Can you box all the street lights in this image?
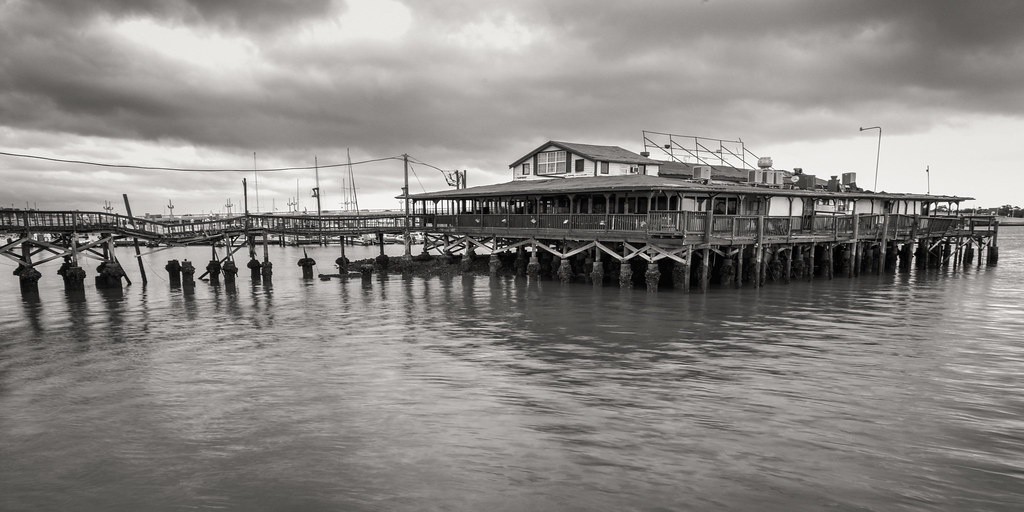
[860,126,882,231]
